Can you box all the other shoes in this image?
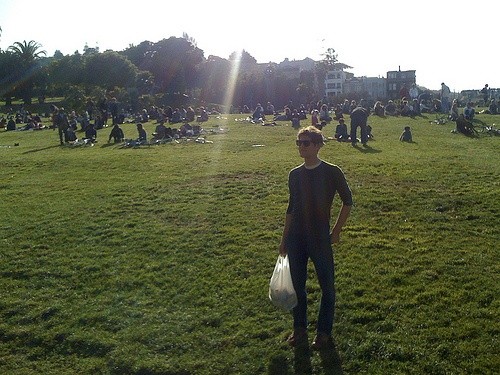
[287,332,308,345]
[312,335,329,349]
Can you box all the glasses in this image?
[296,140,312,147]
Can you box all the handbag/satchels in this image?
[269,254,298,312]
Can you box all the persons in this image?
[398,126,413,143]
[0,96,219,144]
[348,107,368,146]
[234,83,500,130]
[455,115,479,136]
[363,125,375,140]
[334,119,360,142]
[279,127,352,352]
